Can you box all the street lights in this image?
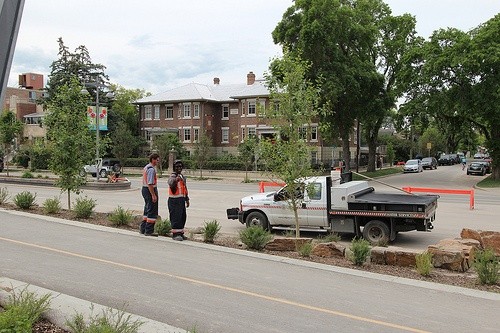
[78,75,115,180]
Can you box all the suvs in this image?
[78,157,123,178]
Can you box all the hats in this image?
[173,160,183,168]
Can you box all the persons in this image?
[167,160,190,241]
[139,154,160,237]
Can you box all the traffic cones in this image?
[259,181,266,193]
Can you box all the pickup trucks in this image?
[227,175,441,247]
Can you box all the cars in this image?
[437,151,494,174]
[403,158,423,173]
[421,156,438,170]
[467,162,487,176]
[393,160,406,166]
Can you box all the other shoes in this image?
[182,234,187,240]
[141,230,144,234]
[144,232,158,236]
[172,233,183,241]
